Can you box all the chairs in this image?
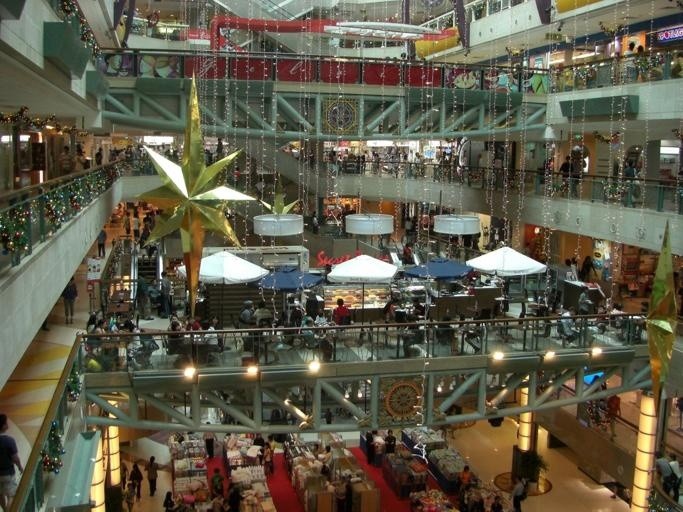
[79,280,643,373]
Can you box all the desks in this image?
[371,427,515,512]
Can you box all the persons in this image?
[62,276,78,324]
[331,475,353,512]
[365,430,396,465]
[120,454,175,511]
[623,43,648,83]
[586,64,597,89]
[623,158,638,208]
[86,271,481,371]
[325,408,333,425]
[364,151,423,175]
[211,468,244,512]
[459,464,524,511]
[605,394,621,437]
[165,149,179,165]
[2,413,23,512]
[203,422,218,459]
[58,146,142,173]
[313,442,333,465]
[405,216,418,236]
[558,156,582,198]
[312,211,319,234]
[610,480,629,504]
[654,452,682,504]
[560,254,649,345]
[123,210,159,241]
[253,433,275,474]
[216,138,223,158]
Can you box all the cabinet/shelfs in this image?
[161,424,381,512]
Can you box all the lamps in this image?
[432,215,481,238]
[344,210,394,236]
[251,210,303,237]
